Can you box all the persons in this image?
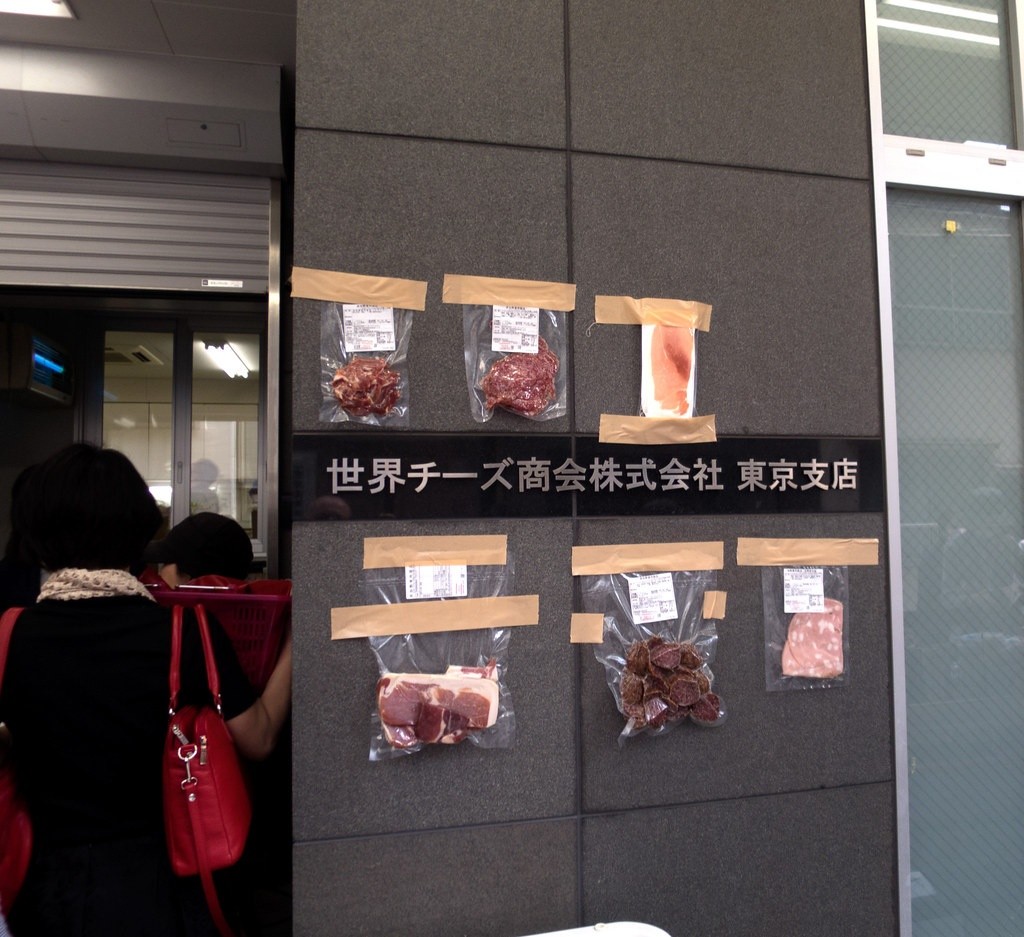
[141,512,256,608]
[0,442,294,937]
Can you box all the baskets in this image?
[149,578,292,687]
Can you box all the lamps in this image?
[204,340,250,380]
[12,323,74,408]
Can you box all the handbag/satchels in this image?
[0,605,32,919]
[164,604,251,877]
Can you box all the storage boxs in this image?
[153,579,292,688]
